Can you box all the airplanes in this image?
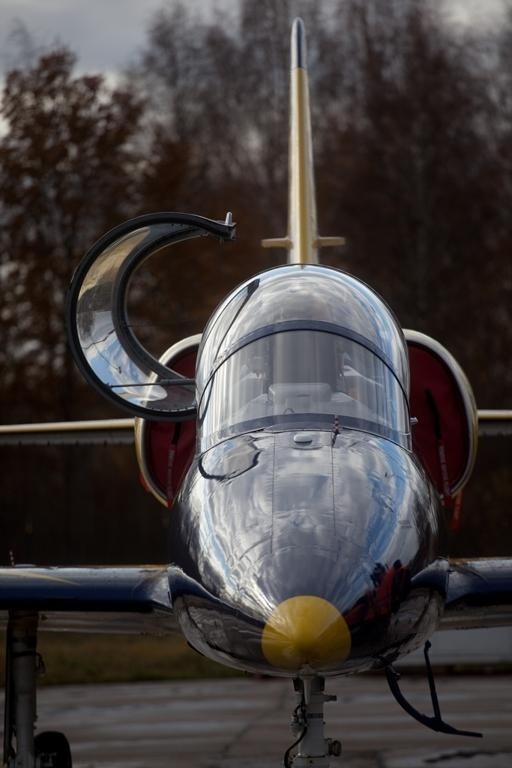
[0,16,512,767]
